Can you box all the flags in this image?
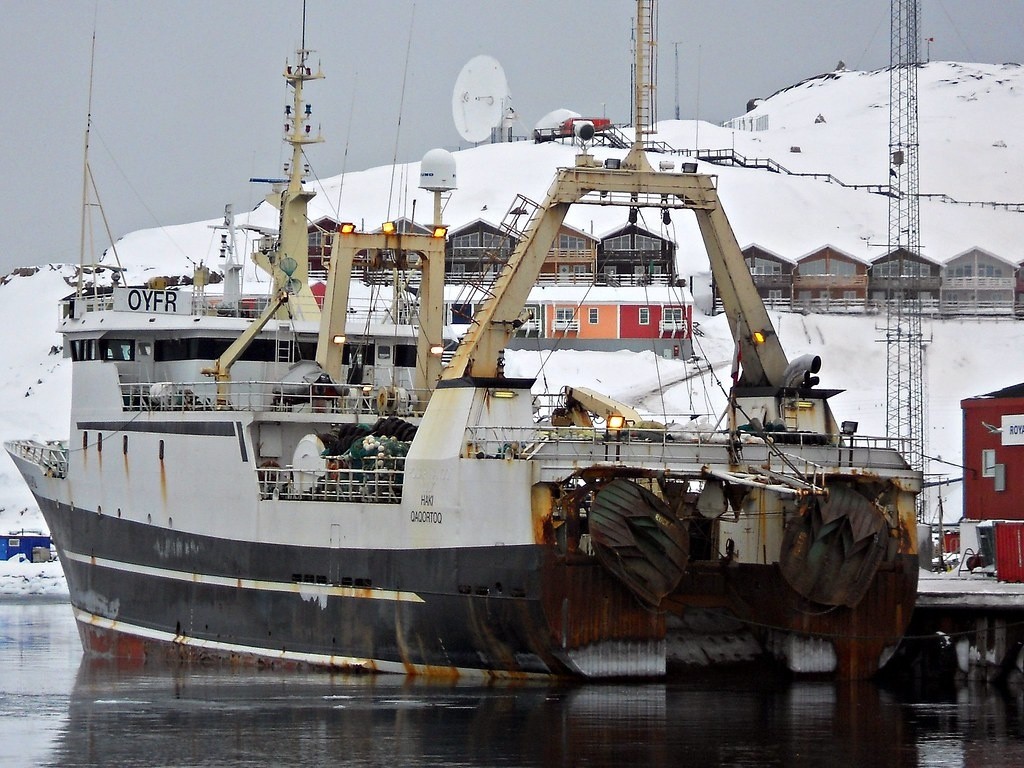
[730,315,742,386]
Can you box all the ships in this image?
[2,1,924,686]
